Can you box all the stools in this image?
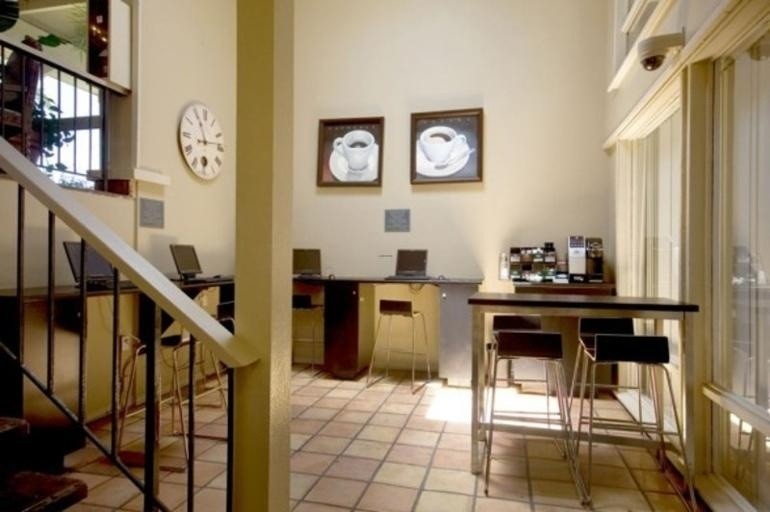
[478,326,585,506]
[569,316,666,459]
[480,316,566,460]
[573,335,699,512]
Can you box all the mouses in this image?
[329,274,335,280]
[438,275,445,282]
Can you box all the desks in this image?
[343,277,483,383]
[292,278,341,381]
[3,276,236,454]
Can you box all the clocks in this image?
[178,102,226,182]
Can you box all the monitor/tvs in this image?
[293,248,322,280]
[63,241,119,292]
[170,244,203,278]
[396,249,428,276]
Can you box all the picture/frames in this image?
[409,106,484,185]
[316,116,384,188]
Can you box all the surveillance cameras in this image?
[636,31,687,72]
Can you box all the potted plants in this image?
[30,93,76,163]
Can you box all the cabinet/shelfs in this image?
[513,278,616,394]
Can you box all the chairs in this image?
[171,301,237,409]
[0,38,42,161]
[365,300,433,393]
[293,293,325,376]
[118,327,232,473]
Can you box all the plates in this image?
[416,139,470,177]
[328,143,379,182]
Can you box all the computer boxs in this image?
[586,237,606,285]
[567,234,587,285]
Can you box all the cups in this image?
[419,126,467,168]
[333,129,375,173]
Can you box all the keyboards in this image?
[385,276,431,280]
[184,275,234,285]
[103,278,137,290]
